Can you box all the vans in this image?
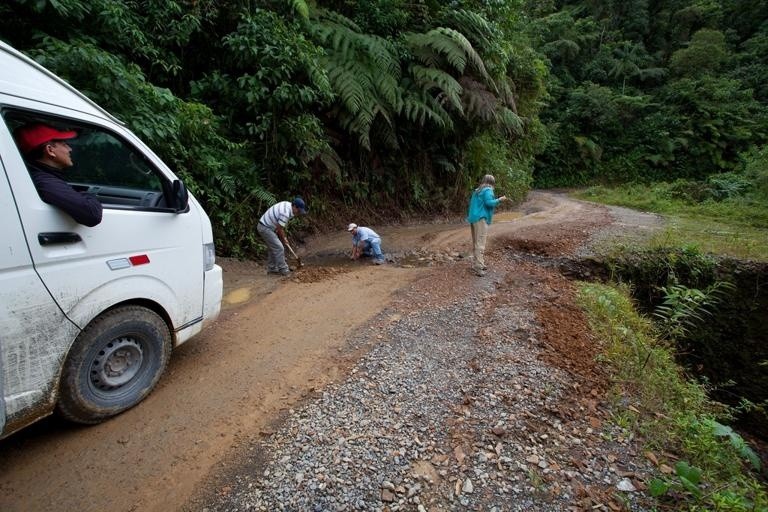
[0,40,222,441]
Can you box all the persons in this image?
[348,223,384,265]
[257,198,307,275]
[464,175,507,277]
[14,122,102,227]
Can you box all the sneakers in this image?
[376,259,384,265]
[279,268,294,275]
[267,267,280,274]
[471,265,486,277]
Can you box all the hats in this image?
[292,198,307,216]
[347,223,358,231]
[13,122,79,152]
